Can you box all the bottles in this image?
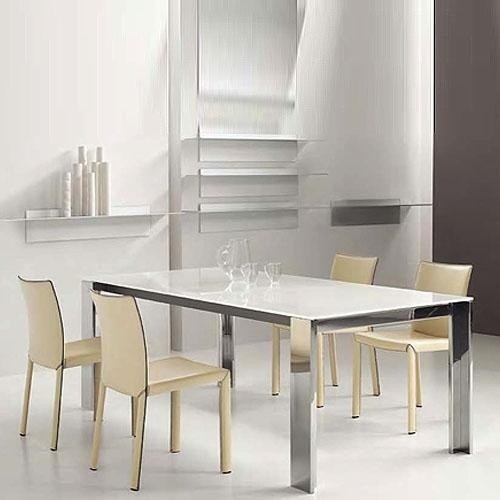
[72,145,111,216]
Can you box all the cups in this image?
[218,239,283,294]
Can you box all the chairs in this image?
[87,286,233,492]
[14,272,140,452]
[268,251,471,437]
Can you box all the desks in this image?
[80,264,481,495]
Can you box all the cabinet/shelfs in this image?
[197,133,332,215]
